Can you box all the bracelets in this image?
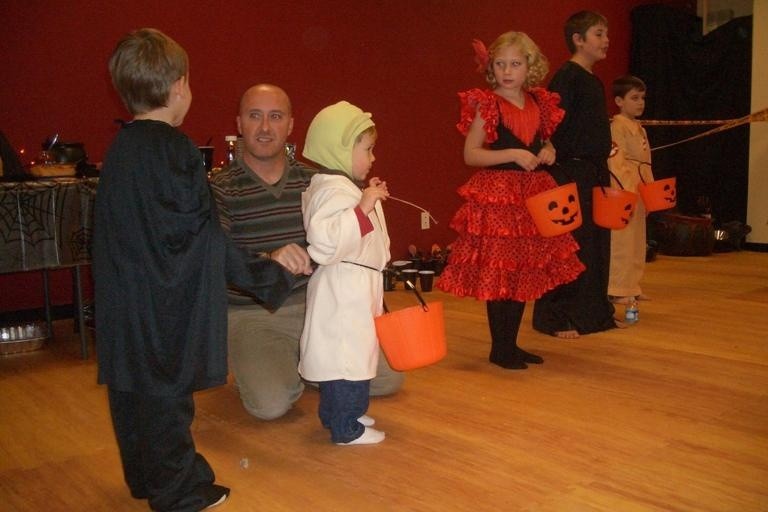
[270,250,272,259]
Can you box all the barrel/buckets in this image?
[591,167,641,234]
[519,161,584,239]
[639,158,679,214]
[375,266,450,371]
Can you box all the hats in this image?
[302,99,375,182]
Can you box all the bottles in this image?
[624,296,639,324]
[224,135,237,165]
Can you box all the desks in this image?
[0,177,98,361]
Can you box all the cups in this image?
[392,259,434,292]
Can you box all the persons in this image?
[607,74,655,305]
[435,33,588,369]
[210,84,406,420]
[531,10,628,339]
[301,101,392,446]
[91,29,296,512]
